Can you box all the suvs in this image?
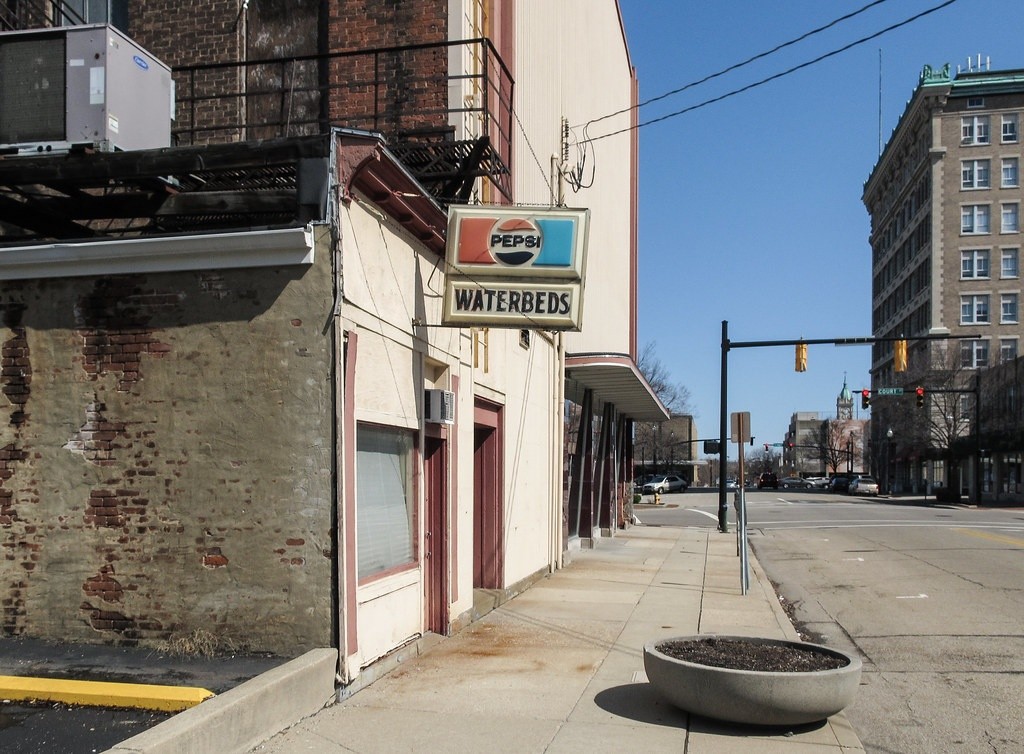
[756,473,779,490]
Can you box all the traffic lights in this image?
[915,387,924,410]
[765,444,768,451]
[789,443,792,451]
[862,389,870,410]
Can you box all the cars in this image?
[806,474,857,493]
[726,479,751,489]
[848,478,879,497]
[778,476,816,489]
[643,475,688,495]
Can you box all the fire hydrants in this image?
[654,492,661,504]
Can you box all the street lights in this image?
[886,427,894,495]
[846,439,849,473]
[670,431,674,475]
[651,424,657,477]
